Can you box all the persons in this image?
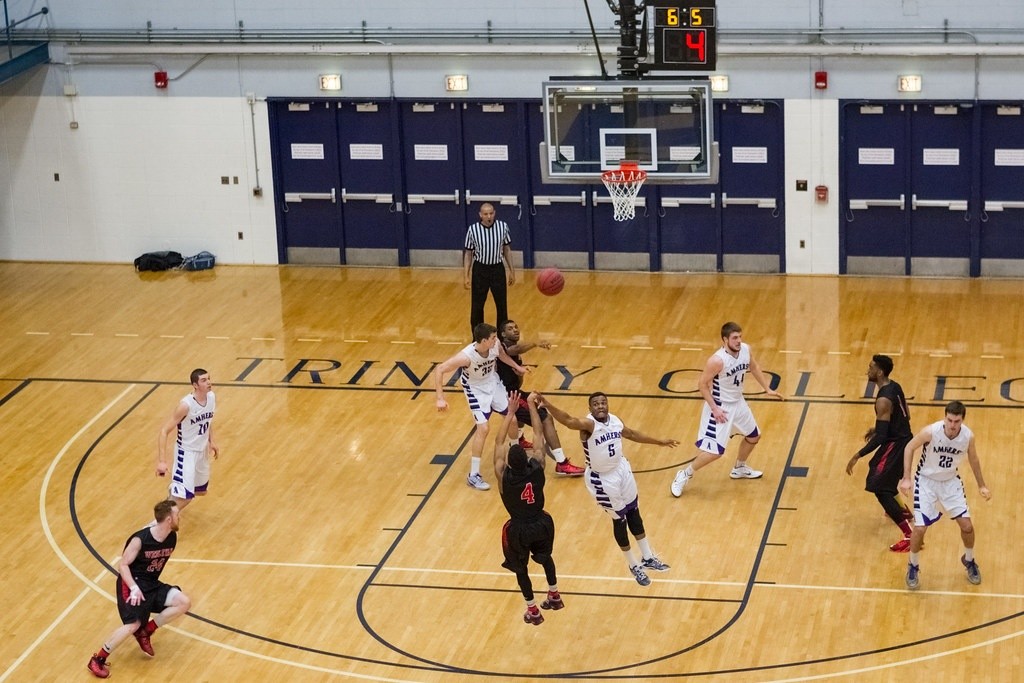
[435,323,526,490]
[146,369,218,528]
[533,389,681,586]
[493,390,566,626]
[88,500,191,679]
[845,355,925,552]
[670,321,784,497]
[495,320,585,477]
[899,401,992,589]
[463,203,516,344]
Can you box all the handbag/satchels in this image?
[182,251,216,271]
[134,251,181,271]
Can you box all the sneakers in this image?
[671,469,693,497]
[890,535,909,552]
[508,433,533,449]
[133,628,154,658]
[961,553,981,584]
[540,594,564,611]
[730,466,763,479]
[629,564,651,586]
[555,458,585,476]
[467,473,491,490]
[886,504,914,522]
[641,555,669,572]
[906,561,919,588]
[524,610,544,626]
[88,653,110,678]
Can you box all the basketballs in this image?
[537,268,565,296]
